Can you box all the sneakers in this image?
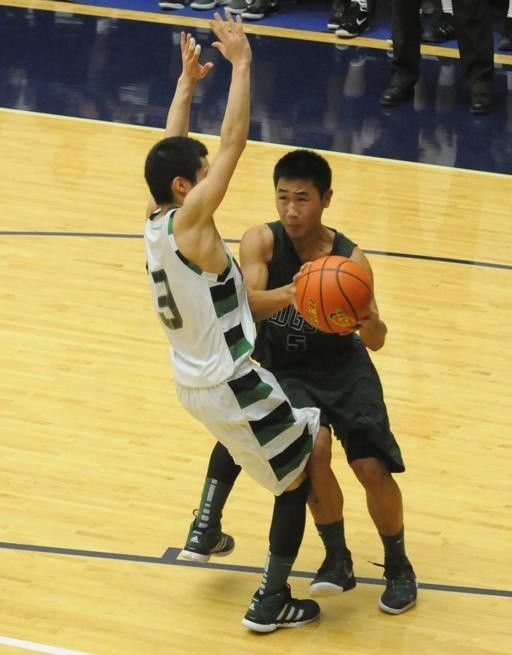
[241,584,320,632]
[378,570,417,614]
[183,520,234,560]
[421,12,457,43]
[158,0,281,19]
[327,0,371,37]
[310,549,356,593]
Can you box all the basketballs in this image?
[296,256,373,333]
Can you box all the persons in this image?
[142,10,323,636]
[237,148,422,613]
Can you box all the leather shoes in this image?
[379,85,415,105]
[468,92,496,114]
